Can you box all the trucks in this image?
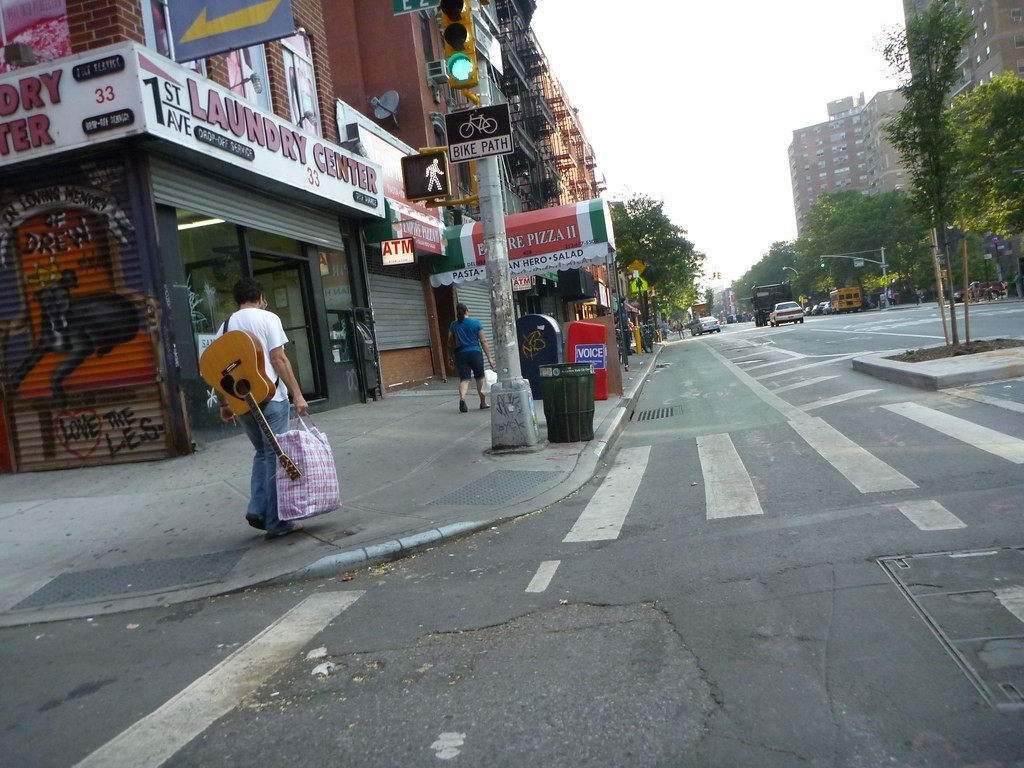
[830,287,862,314]
[692,303,711,321]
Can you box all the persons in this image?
[1012,271,1023,298]
[447,303,493,412]
[661,320,668,339]
[972,282,980,302]
[915,288,921,305]
[677,321,685,339]
[215,278,309,539]
[628,318,634,340]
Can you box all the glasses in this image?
[263,300,267,309]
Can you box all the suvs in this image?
[953,281,1006,302]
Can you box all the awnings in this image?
[427,198,629,375]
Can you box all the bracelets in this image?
[219,404,229,407]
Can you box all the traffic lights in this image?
[400,151,453,202]
[820,259,825,269]
[717,272,721,279]
[438,1,479,89]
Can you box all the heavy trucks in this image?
[749,279,794,327]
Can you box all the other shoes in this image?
[460,399,467,412]
[480,403,490,409]
[265,520,303,538]
[245,513,266,531]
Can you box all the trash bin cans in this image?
[515,313,563,400]
[538,361,596,443]
[565,320,608,400]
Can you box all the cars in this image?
[819,301,832,315]
[691,316,720,336]
[769,301,804,327]
[811,304,820,315]
[804,306,811,316]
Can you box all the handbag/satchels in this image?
[275,411,342,521]
[480,366,497,396]
[448,322,460,348]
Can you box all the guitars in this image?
[200,329,301,481]
